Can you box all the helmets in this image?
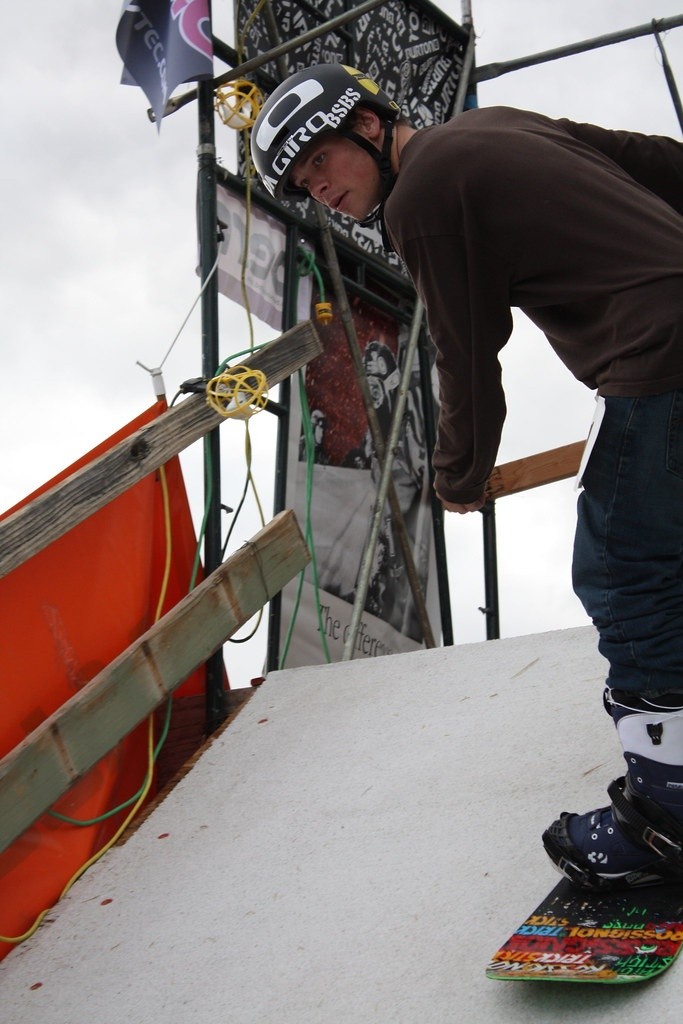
[252,63,401,203]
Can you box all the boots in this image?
[542,687,683,888]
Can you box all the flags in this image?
[116,0,213,134]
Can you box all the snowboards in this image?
[479,876,683,991]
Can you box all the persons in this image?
[251,64,683,891]
